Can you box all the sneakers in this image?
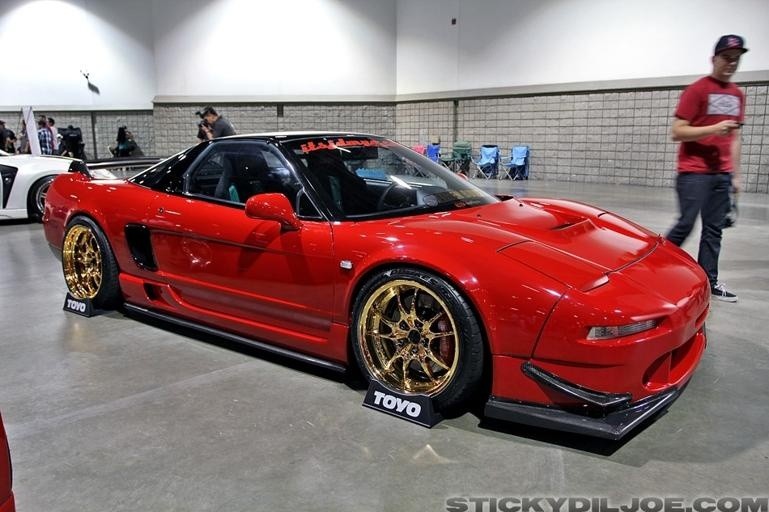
[710,284,739,303]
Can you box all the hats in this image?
[714,35,749,55]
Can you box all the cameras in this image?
[195,111,209,128]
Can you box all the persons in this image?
[662,34,750,303]
[109,126,143,158]
[199,108,237,141]
[0,115,87,162]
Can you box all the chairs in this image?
[384,140,472,179]
[224,149,284,205]
[304,146,376,220]
[499,144,531,180]
[468,144,499,179]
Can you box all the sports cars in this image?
[41,130,712,444]
[0,148,88,223]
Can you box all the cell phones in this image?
[735,121,744,126]
